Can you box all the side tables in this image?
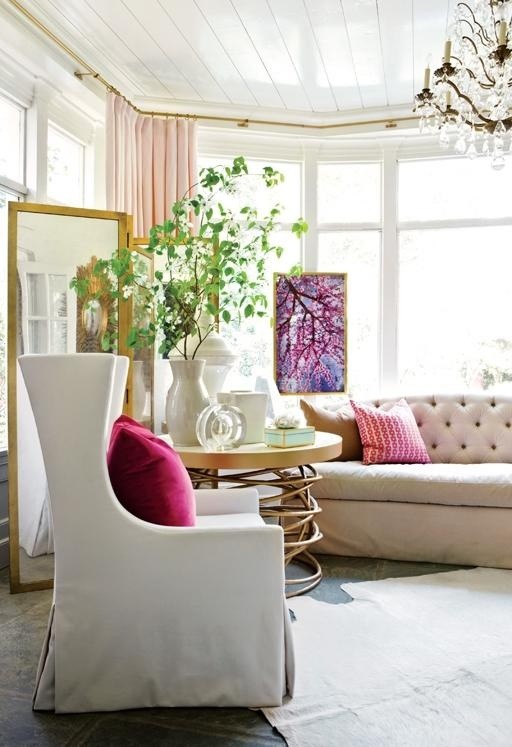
[157,429,344,599]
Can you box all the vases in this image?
[162,359,223,447]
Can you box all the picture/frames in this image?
[273,271,348,395]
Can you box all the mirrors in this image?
[7,204,133,590]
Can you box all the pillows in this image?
[350,400,431,463]
[108,415,196,528]
[298,399,362,464]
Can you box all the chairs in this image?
[18,352,293,712]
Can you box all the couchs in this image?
[288,394,511,567]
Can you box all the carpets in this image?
[248,567,512,747]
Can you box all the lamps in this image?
[410,0,512,155]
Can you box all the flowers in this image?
[68,154,309,358]
